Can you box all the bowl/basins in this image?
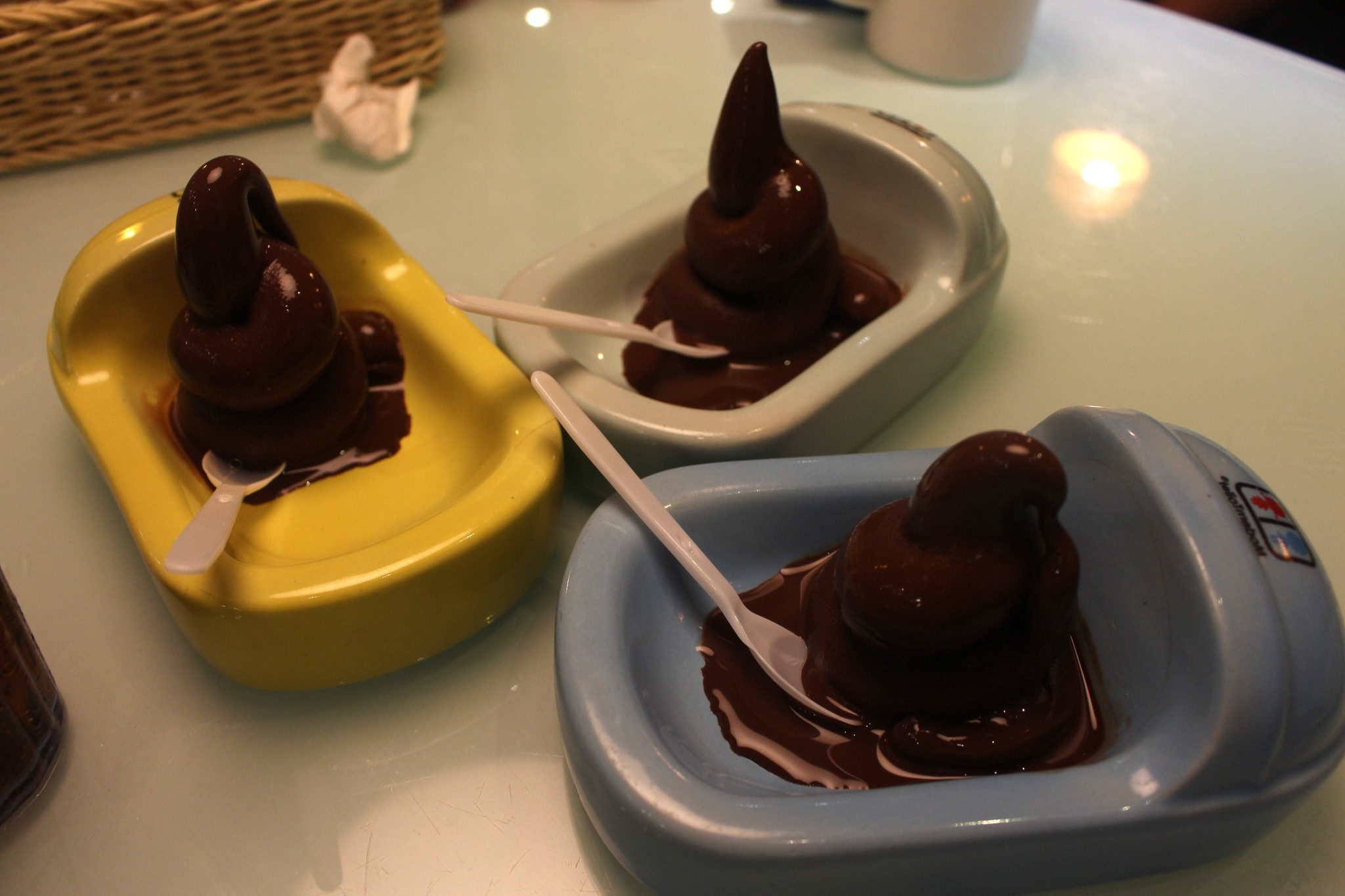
[488,102,1011,488]
[555,404,1345,896]
[41,170,563,688]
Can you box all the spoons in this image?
[532,372,860,728]
[165,452,287,578]
[447,290,727,359]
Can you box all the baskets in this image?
[0,0,450,177]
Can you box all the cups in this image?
[830,0,1037,84]
[0,572,69,829]
